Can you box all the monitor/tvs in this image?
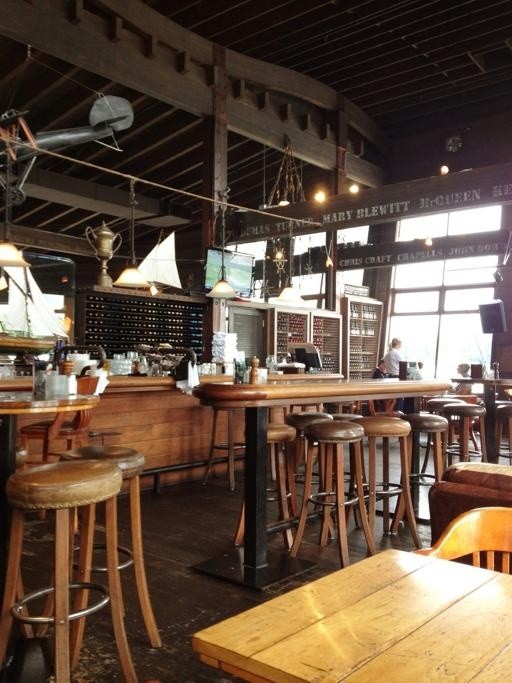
[202,246,255,298]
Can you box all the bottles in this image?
[348,301,382,381]
[277,313,307,366]
[312,314,340,374]
[84,295,204,361]
[492,361,500,380]
[36,336,95,400]
[232,354,260,385]
[13,349,34,377]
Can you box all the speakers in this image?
[479,301,507,333]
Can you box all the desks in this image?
[190,380,462,593]
[452,374,512,475]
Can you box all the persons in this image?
[373,359,391,408]
[385,337,406,411]
[449,362,472,395]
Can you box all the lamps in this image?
[205,202,239,298]
[112,178,151,289]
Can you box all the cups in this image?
[399,360,408,380]
[408,361,417,380]
[107,350,176,377]
[197,361,217,376]
[486,370,495,380]
[265,354,277,374]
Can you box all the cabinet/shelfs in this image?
[310,312,343,373]
[269,306,310,364]
[76,291,208,365]
[341,297,384,379]
